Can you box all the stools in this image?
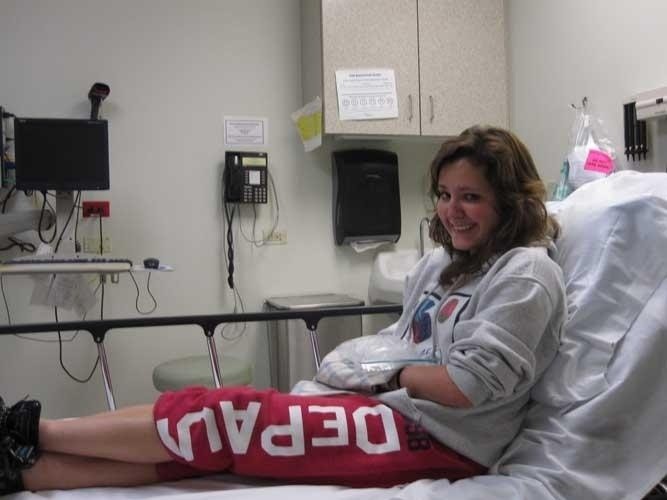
[151,354,252,394]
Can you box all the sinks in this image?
[370,251,427,304]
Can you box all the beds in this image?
[0,170,667,500]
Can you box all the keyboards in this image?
[2,257,133,267]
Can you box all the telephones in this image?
[224,150,270,206]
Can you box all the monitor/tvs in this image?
[14,116,110,191]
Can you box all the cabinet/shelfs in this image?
[300,0,512,135]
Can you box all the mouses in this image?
[143,257,160,269]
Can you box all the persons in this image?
[0,123,568,495]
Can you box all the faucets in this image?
[418,218,432,257]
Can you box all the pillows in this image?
[493,168,667,400]
